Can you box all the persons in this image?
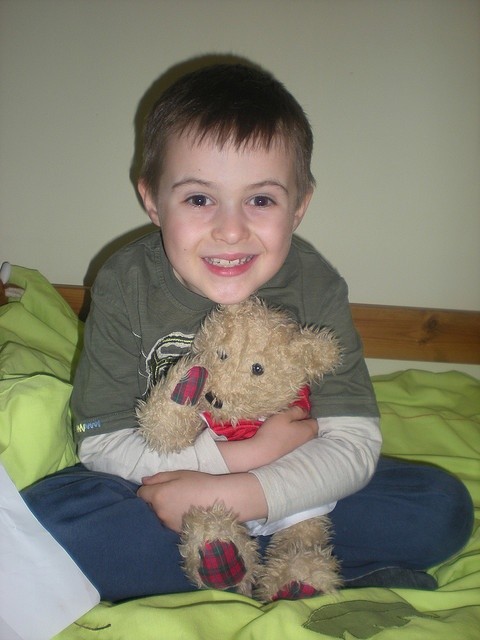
[16,50,476,609]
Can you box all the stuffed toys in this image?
[132,294,345,604]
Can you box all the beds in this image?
[0,261,480,640]
